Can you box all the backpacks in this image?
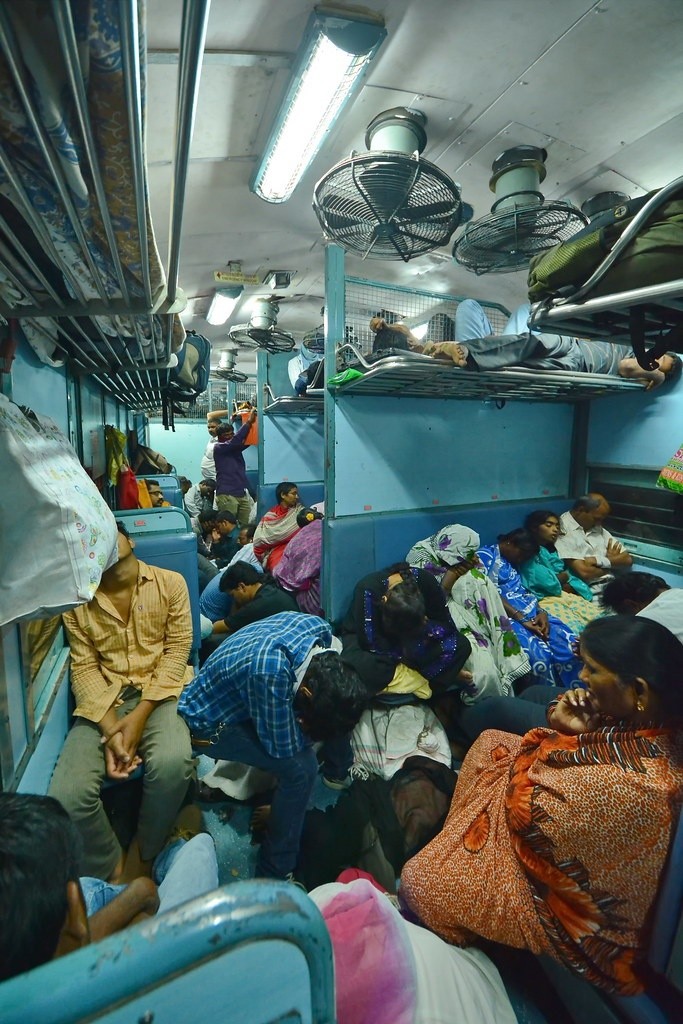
[166,330,211,401]
[296,361,324,395]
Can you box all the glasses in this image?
[224,432,234,439]
[150,491,162,496]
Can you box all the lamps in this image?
[249,3,388,205]
[206,284,244,326]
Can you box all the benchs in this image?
[245,469,324,525]
[325,494,579,632]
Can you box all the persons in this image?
[0,793,160,985]
[370,298,683,391]
[477,527,590,692]
[272,508,322,617]
[237,524,257,547]
[177,610,365,881]
[401,616,683,996]
[518,510,617,639]
[202,561,300,660]
[135,410,325,625]
[553,493,634,602]
[407,523,532,704]
[253,482,307,576]
[200,613,215,640]
[598,571,683,646]
[137,478,171,509]
[340,562,472,705]
[46,521,193,885]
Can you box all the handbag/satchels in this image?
[104,426,139,509]
[327,368,363,385]
[0,396,119,624]
[242,413,258,445]
[526,183,683,305]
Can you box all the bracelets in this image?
[512,608,548,624]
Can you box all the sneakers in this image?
[282,873,308,893]
[164,805,203,848]
[321,761,380,789]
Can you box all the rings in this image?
[574,688,580,700]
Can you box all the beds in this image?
[526,174,683,356]
[230,409,259,420]
[261,382,324,415]
[336,342,644,400]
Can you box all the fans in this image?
[311,106,462,263]
[209,349,248,384]
[452,144,592,276]
[300,306,363,356]
[226,298,295,355]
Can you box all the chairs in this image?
[134,474,183,508]
[112,506,201,779]
[0,879,337,1024]
